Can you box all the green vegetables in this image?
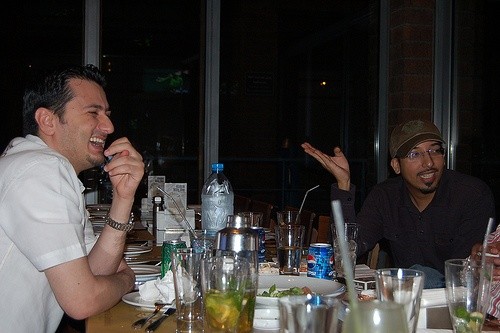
[455,307,482,333]
[225,275,305,328]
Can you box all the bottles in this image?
[201,163,234,230]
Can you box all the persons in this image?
[302,119,496,289]
[469,241,500,283]
[0,63,145,333]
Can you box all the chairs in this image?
[233,194,328,242]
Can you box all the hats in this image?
[388,119,443,159]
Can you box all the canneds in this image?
[306,242,335,282]
[161,238,188,278]
[248,226,265,262]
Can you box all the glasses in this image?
[394,147,444,161]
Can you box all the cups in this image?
[171,210,359,333]
[278,258,494,333]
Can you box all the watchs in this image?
[105,209,136,233]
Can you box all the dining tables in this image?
[86,237,345,333]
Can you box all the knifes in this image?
[145,300,176,333]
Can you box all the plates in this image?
[123,246,153,254]
[256,275,345,305]
[91,220,107,227]
[122,292,197,308]
[129,265,162,278]
